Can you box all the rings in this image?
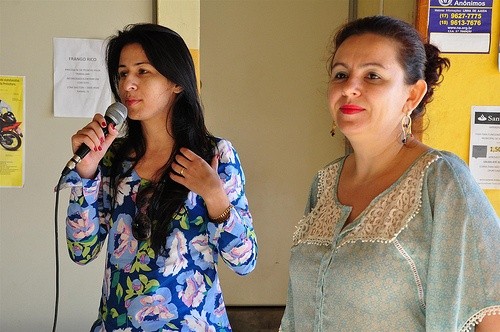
[180,167,186,177]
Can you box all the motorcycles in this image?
[0,111,22,151]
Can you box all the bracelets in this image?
[208,205,234,223]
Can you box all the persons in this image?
[57,22,258,331]
[275,16,500,332]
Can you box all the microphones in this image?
[61,101,128,175]
